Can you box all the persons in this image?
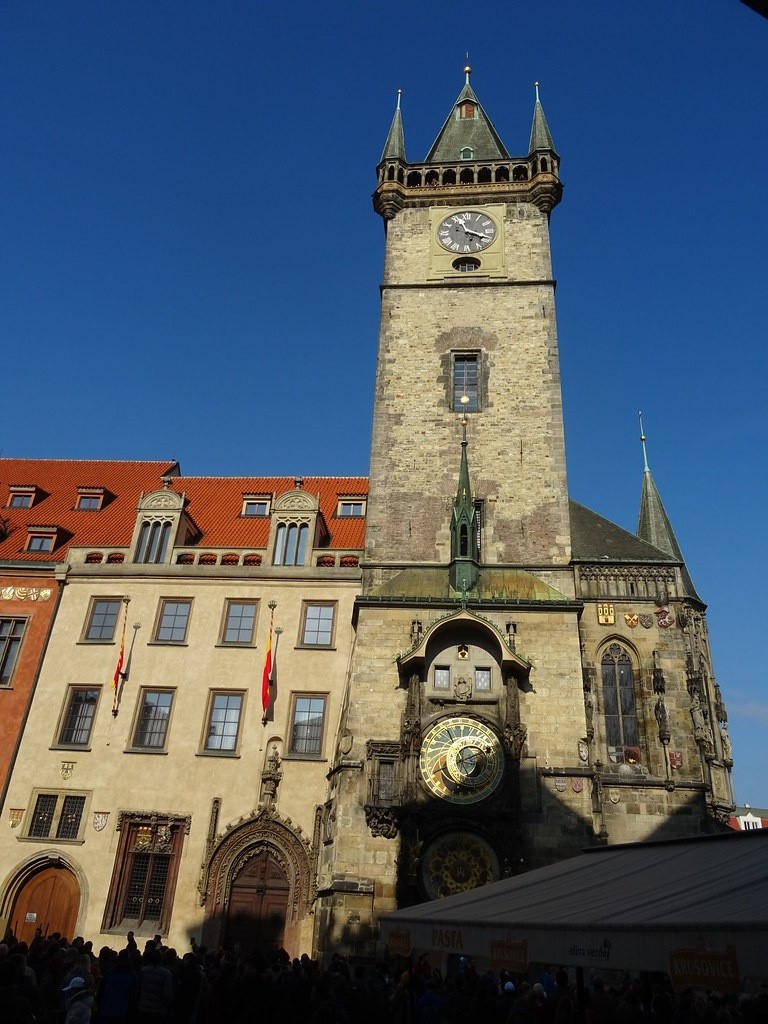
[0,925,768,1024]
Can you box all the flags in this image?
[262,626,272,717]
[113,605,128,697]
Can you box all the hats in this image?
[533,983,544,993]
[504,981,515,992]
[61,976,85,991]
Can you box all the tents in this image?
[376,826,767,986]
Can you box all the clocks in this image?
[434,207,500,254]
